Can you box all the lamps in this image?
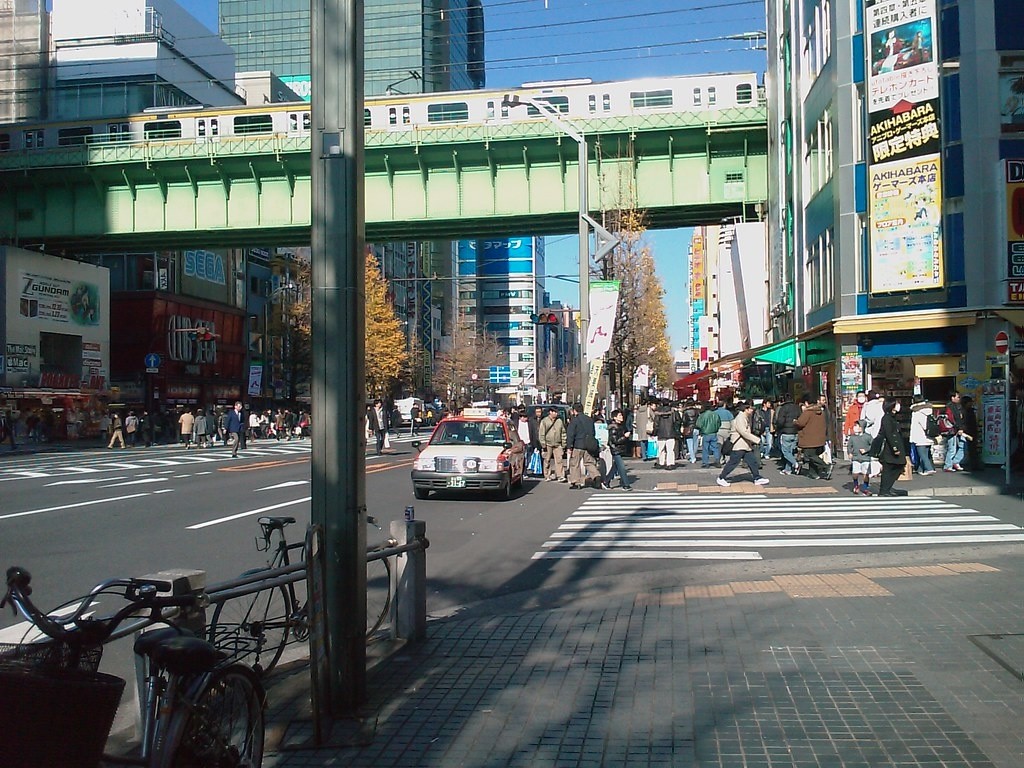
[22,243,46,252]
[46,248,67,257]
[67,255,84,263]
[719,215,743,246]
[95,258,103,268]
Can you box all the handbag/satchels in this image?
[212,424,217,432]
[531,448,543,474]
[586,435,599,455]
[910,443,919,464]
[721,438,732,455]
[648,436,659,458]
[384,432,391,449]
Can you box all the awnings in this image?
[710,337,796,373]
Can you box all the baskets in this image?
[0,640,125,768]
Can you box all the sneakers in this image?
[754,477,770,486]
[716,477,731,486]
[862,490,873,496]
[853,484,859,494]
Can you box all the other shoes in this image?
[807,475,821,479]
[826,464,834,479]
[524,473,633,492]
[878,490,898,496]
[377,451,382,455]
[780,469,792,476]
[795,463,802,475]
[641,454,771,470]
[915,463,963,475]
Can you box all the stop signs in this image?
[995,331,1009,354]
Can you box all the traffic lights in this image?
[530,313,558,325]
[188,331,215,341]
[602,363,609,375]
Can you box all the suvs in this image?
[525,404,571,427]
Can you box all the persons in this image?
[909,394,936,475]
[844,391,867,474]
[847,420,874,496]
[249,407,311,443]
[448,404,633,491]
[0,409,20,451]
[860,390,885,439]
[366,399,423,456]
[943,389,968,472]
[876,396,906,497]
[1001,382,1024,471]
[911,31,925,63]
[882,26,903,58]
[960,396,984,470]
[632,396,834,487]
[26,409,226,450]
[223,401,245,457]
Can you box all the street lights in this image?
[504,95,621,408]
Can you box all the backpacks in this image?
[919,410,940,437]
[939,403,955,437]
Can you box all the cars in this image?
[410,415,526,498]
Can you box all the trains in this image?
[0,70,766,169]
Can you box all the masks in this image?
[858,397,866,403]
[894,404,900,412]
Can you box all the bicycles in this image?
[209,515,392,677]
[0,566,267,768]
